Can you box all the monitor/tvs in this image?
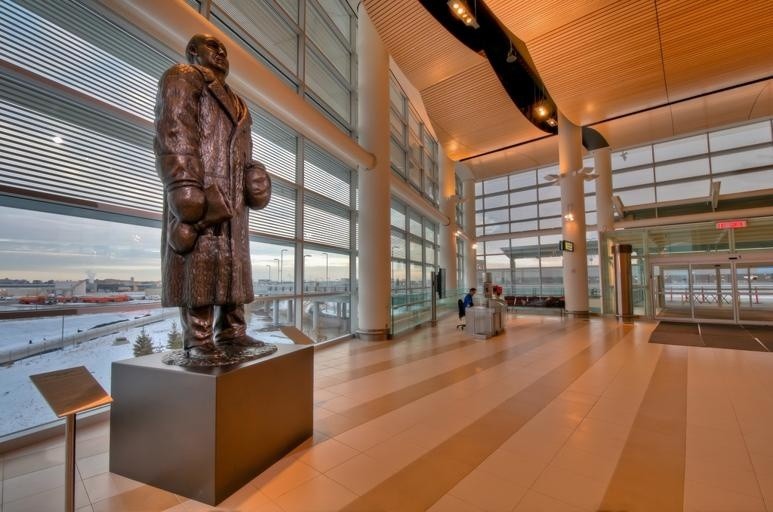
[437,268,448,299]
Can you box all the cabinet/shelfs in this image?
[467,302,506,340]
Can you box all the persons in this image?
[154,33,270,361]
[464,287,477,309]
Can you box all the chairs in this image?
[457,299,466,331]
[504,296,565,318]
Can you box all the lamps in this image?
[542,167,600,185]
[447,0,477,26]
[532,86,549,120]
